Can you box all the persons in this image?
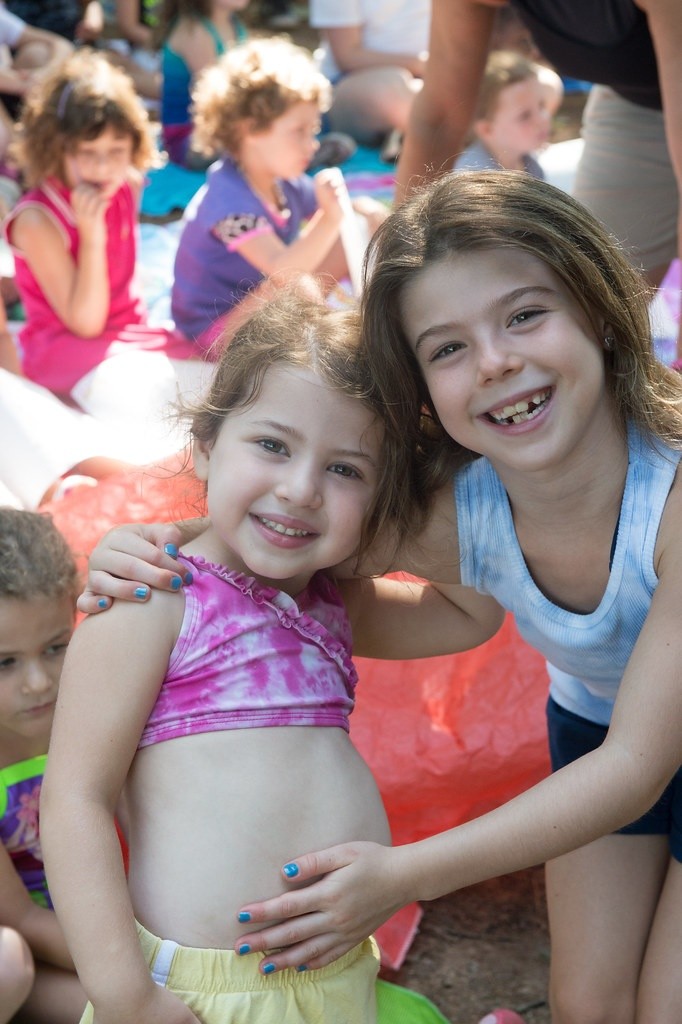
[0,2,680,390]
[0,509,72,1024]
[76,170,682,1024]
[35,304,501,1024]
